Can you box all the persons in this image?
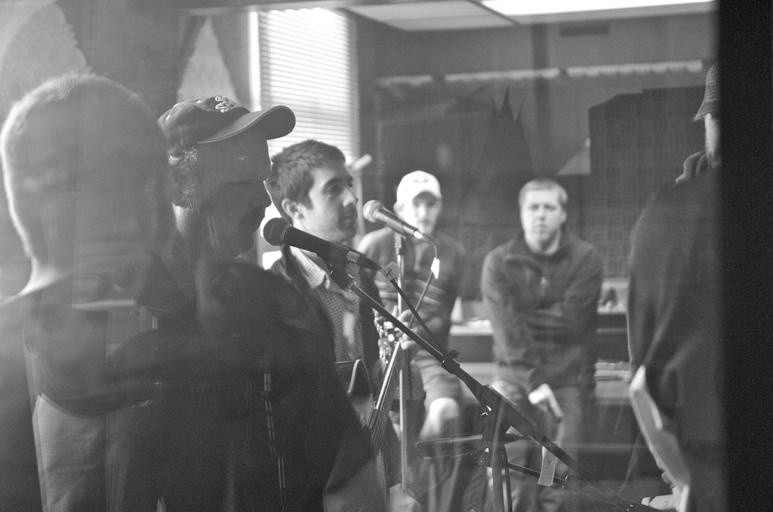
[258,137,403,512]
[155,95,297,261]
[344,170,465,511]
[0,72,392,511]
[624,60,724,511]
[477,177,602,511]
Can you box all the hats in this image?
[157,95,296,145]
[689,61,724,125]
[397,171,441,205]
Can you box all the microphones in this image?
[364,199,430,244]
[261,218,381,276]
